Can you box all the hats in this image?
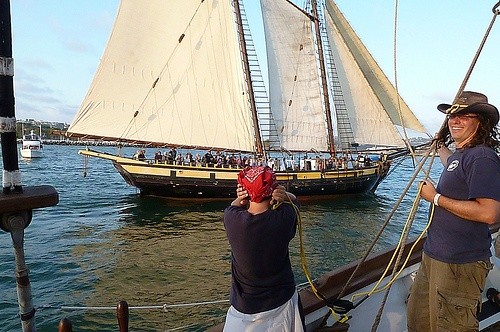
[437,91,500,127]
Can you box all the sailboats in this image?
[64,0,428,198]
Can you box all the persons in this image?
[221,165,307,332]
[407,90,500,332]
[133,148,372,174]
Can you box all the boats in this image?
[19,131,44,158]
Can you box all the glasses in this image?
[446,115,478,120]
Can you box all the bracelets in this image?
[434,194,442,206]
